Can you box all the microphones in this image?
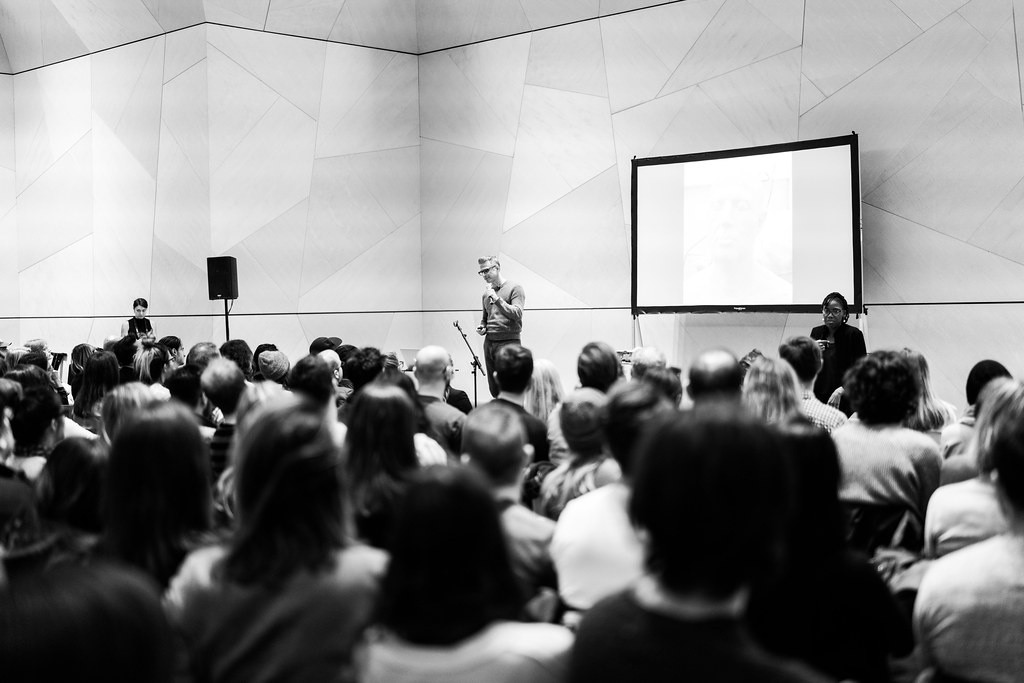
[487,286,494,305]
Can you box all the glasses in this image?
[822,308,844,316]
[340,360,345,367]
[478,265,495,275]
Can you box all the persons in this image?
[121,298,155,341]
[0,338,1023,682]
[809,292,866,420]
[476,255,524,398]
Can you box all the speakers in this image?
[206,256,239,300]
